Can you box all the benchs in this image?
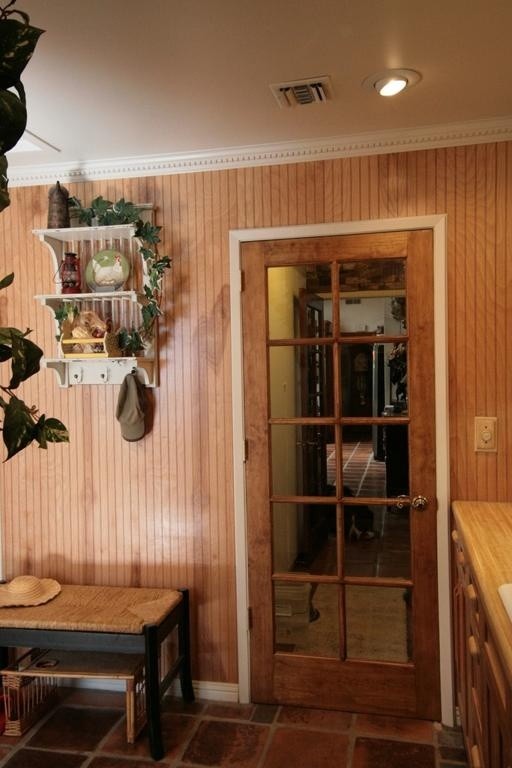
[0,647,149,743]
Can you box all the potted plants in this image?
[76,200,165,353]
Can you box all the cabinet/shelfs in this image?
[383,404,412,510]
[447,501,512,762]
[29,200,159,386]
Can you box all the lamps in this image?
[361,69,423,97]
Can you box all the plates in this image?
[86,250,129,292]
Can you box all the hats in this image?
[118,374,148,442]
[2,577,62,607]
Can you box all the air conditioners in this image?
[270,75,337,109]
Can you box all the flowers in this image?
[387,344,407,360]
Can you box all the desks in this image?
[0,582,198,759]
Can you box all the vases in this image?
[392,397,412,409]
